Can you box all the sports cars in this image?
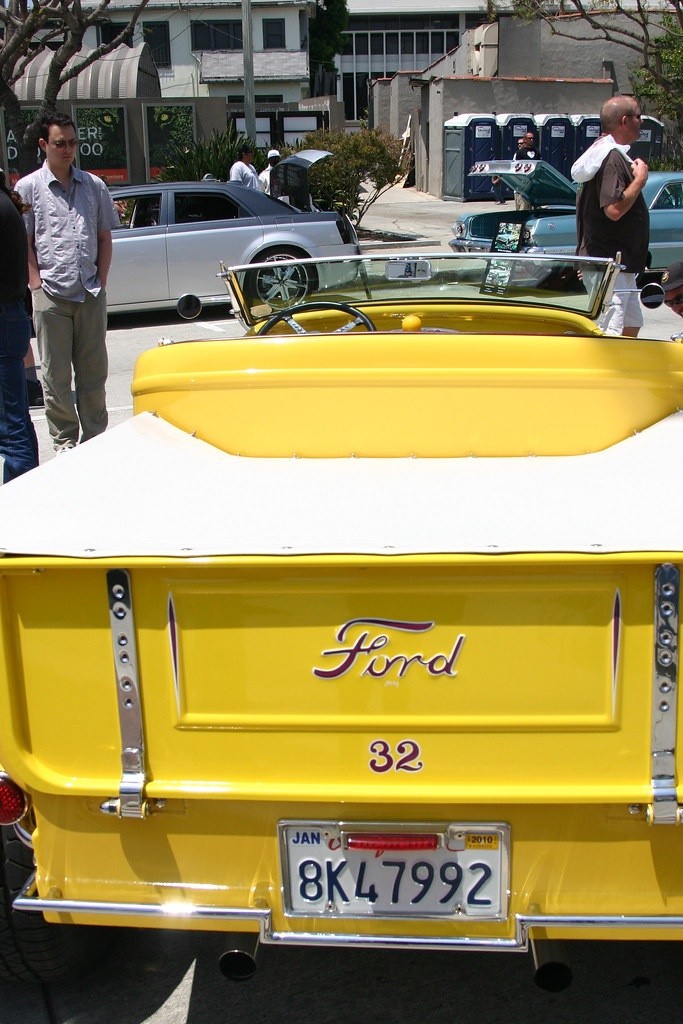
[0,250,683,992]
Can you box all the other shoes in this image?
[27,378,42,402]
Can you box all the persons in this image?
[258,149,290,205]
[511,131,541,210]
[0,167,44,483]
[229,145,260,191]
[491,175,507,205]
[570,96,650,338]
[12,112,120,457]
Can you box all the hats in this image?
[268,149,281,159]
[661,262,683,291]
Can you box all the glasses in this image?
[627,114,642,119]
[517,143,523,145]
[526,137,535,140]
[664,294,683,307]
[48,138,79,148]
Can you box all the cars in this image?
[22,148,364,336]
[448,157,683,291]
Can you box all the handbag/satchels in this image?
[570,134,635,183]
